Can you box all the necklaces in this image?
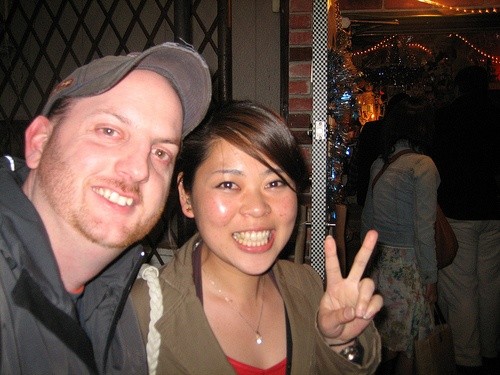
[203,269,269,345]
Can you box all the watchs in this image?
[325,341,362,363]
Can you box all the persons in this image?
[435,66,500,375]
[129,100,383,375]
[361,115,458,375]
[0,44,212,375]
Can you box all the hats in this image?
[42,42,213,140]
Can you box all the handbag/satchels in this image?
[433,203,458,269]
